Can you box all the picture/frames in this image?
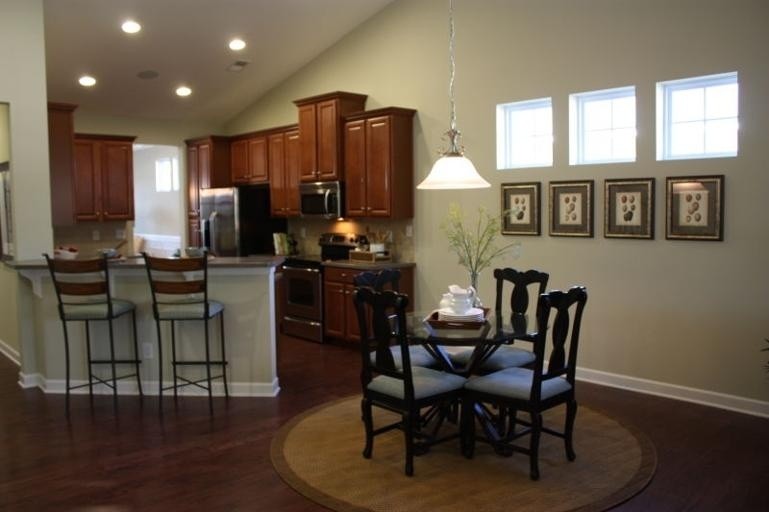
[664,174,724,242]
[500,183,540,237]
[604,178,654,241]
[548,179,595,238]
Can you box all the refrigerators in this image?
[199,184,273,259]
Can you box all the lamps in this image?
[415,0,493,190]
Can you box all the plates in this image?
[436,307,485,325]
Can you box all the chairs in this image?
[352,286,468,478]
[463,285,588,480]
[351,267,459,434]
[141,251,227,416]
[43,253,143,412]
[492,267,549,437]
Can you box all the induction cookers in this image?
[285,232,366,262]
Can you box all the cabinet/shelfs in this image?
[292,90,369,182]
[183,134,232,248]
[321,259,415,352]
[73,135,140,222]
[47,101,80,227]
[341,106,418,223]
[229,129,269,184]
[268,121,299,218]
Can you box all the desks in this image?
[383,307,531,458]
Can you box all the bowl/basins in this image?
[52,249,80,262]
[184,245,208,257]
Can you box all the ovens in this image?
[275,258,326,344]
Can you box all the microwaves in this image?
[298,180,347,220]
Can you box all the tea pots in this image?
[448,283,478,313]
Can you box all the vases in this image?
[469,271,481,294]
